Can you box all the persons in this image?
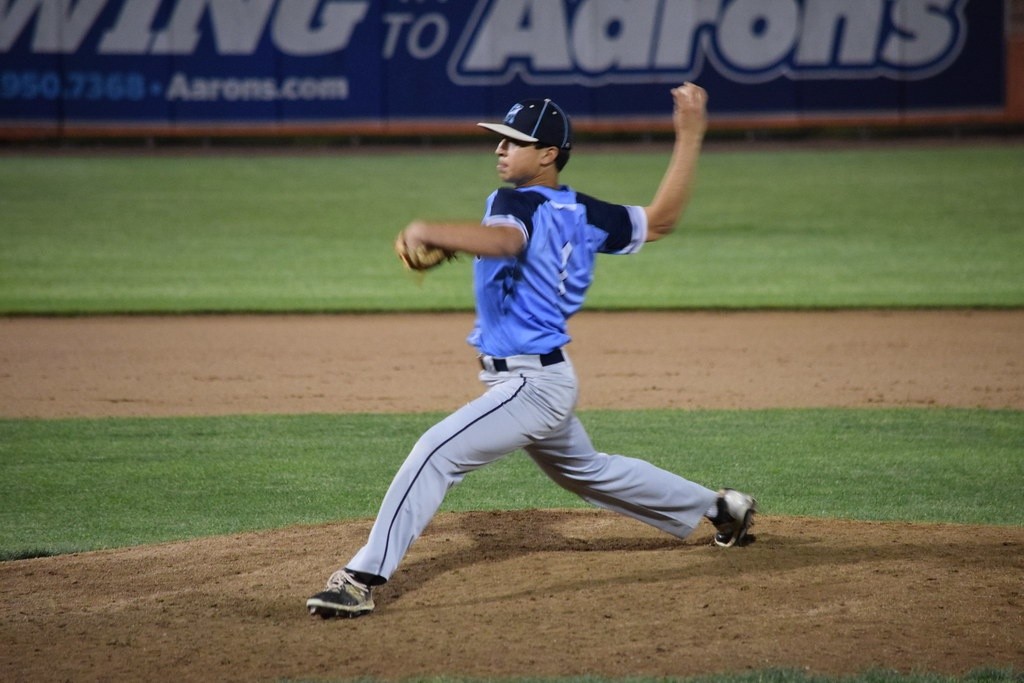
[305,81,758,620]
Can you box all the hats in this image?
[476,98,571,149]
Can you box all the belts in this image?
[477,349,564,373]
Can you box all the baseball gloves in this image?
[394,227,459,272]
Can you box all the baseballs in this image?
[676,85,702,103]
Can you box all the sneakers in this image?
[305,570,375,619]
[705,487,757,547]
